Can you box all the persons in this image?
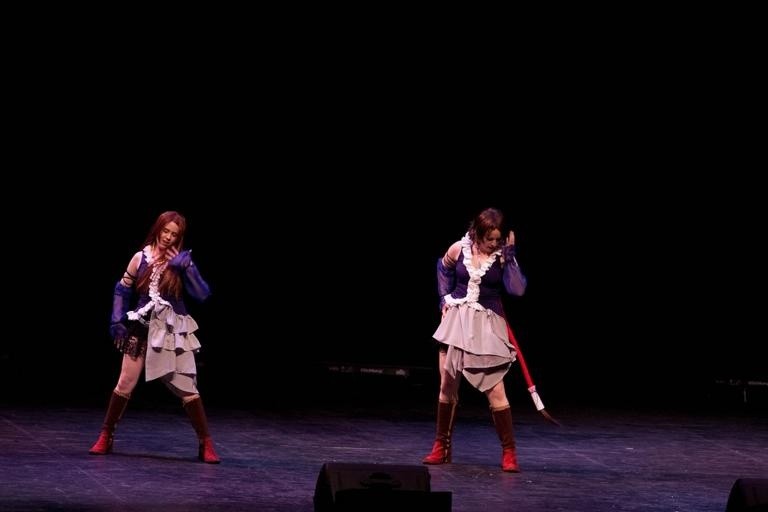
[88,209,222,465]
[420,209,530,473]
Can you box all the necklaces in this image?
[152,242,168,264]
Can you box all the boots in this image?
[420,397,459,466]
[489,403,521,474]
[88,389,132,456]
[182,394,219,464]
